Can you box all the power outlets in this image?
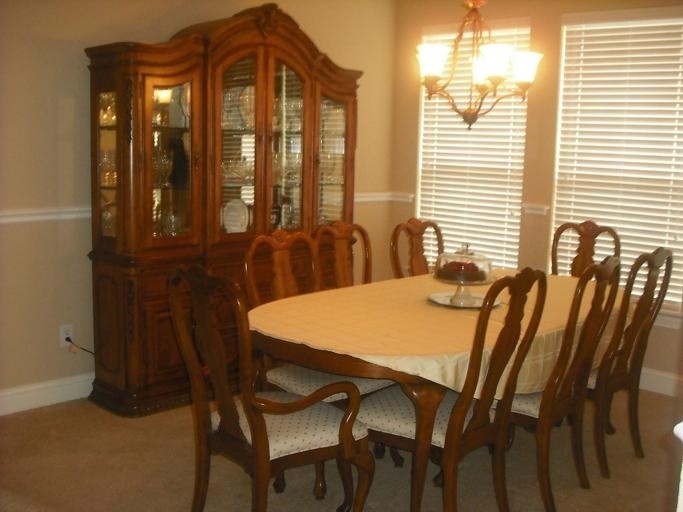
[57,324,75,348]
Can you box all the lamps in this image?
[415,0,545,129]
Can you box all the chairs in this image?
[350,267,547,510]
[244,232,404,498]
[571,246,674,477]
[170,265,374,511]
[552,219,622,274]
[314,219,374,291]
[480,255,627,511]
[390,219,446,278]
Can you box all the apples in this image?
[442,260,479,273]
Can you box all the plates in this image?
[428,293,501,310]
[221,197,249,233]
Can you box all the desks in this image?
[245,271,640,511]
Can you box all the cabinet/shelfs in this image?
[84,2,364,415]
